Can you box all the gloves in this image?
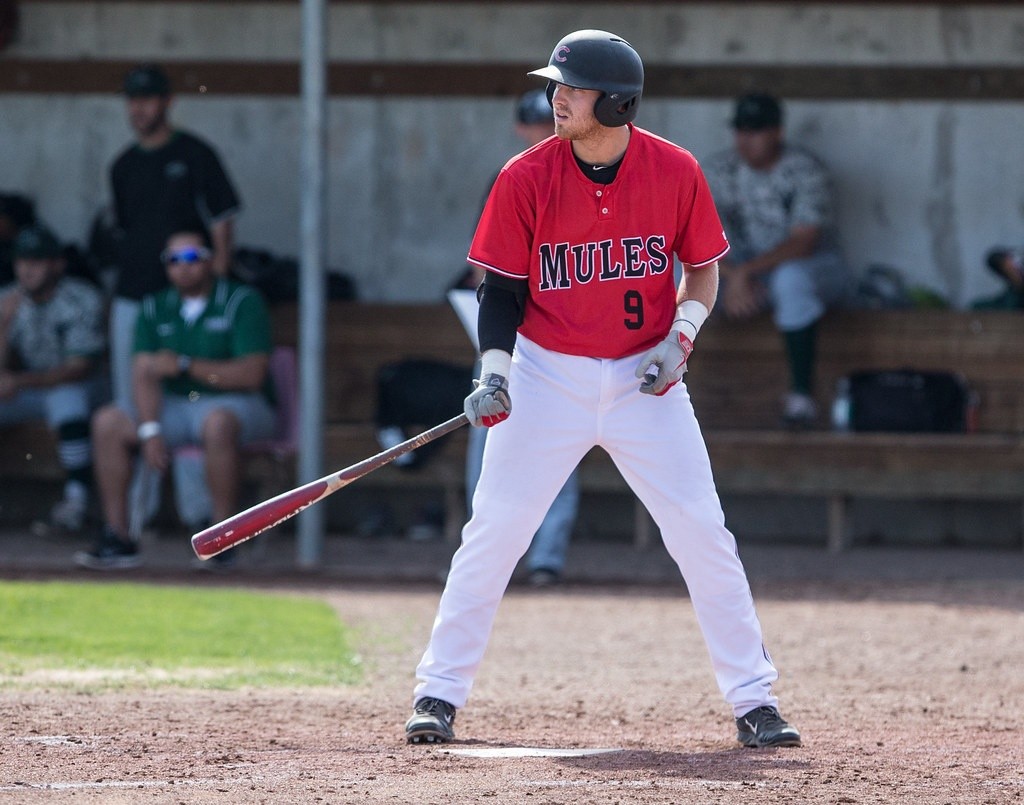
[635,330,694,396]
[464,372,512,427]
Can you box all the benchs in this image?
[270,301,1023,556]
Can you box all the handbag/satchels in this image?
[833,369,968,432]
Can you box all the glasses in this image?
[166,250,206,264]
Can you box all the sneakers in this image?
[736,706,802,747]
[406,697,456,742]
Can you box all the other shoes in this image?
[783,394,818,421]
[531,569,556,586]
[76,534,146,570]
[192,548,236,572]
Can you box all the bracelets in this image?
[137,424,161,440]
[178,354,192,379]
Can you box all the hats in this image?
[15,227,60,261]
[118,67,166,97]
[732,94,779,129]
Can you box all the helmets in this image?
[527,30,644,127]
[517,88,554,125]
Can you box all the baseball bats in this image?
[191,388,510,563]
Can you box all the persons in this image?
[407,30,800,747]
[466,91,578,586]
[0,65,283,572]
[700,93,844,423]
